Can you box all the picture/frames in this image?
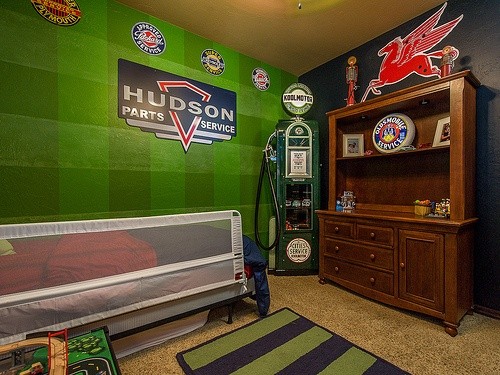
[343,133,364,158]
[430,116,450,147]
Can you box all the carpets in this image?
[175,307,411,375]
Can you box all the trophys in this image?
[439,45,453,78]
[344,55,360,107]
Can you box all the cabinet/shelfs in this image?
[399,220,475,336]
[325,69,481,220]
[315,210,399,307]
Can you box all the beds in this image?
[1,210,271,359]
